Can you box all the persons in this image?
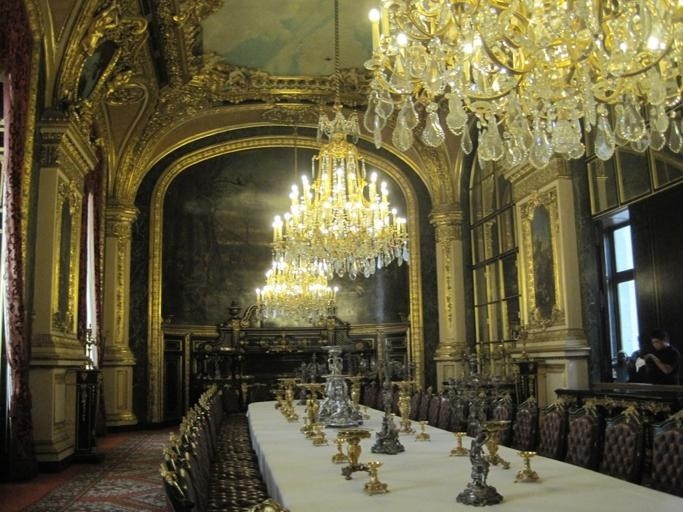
[610,352,631,383]
[628,333,653,381]
[640,328,681,386]
